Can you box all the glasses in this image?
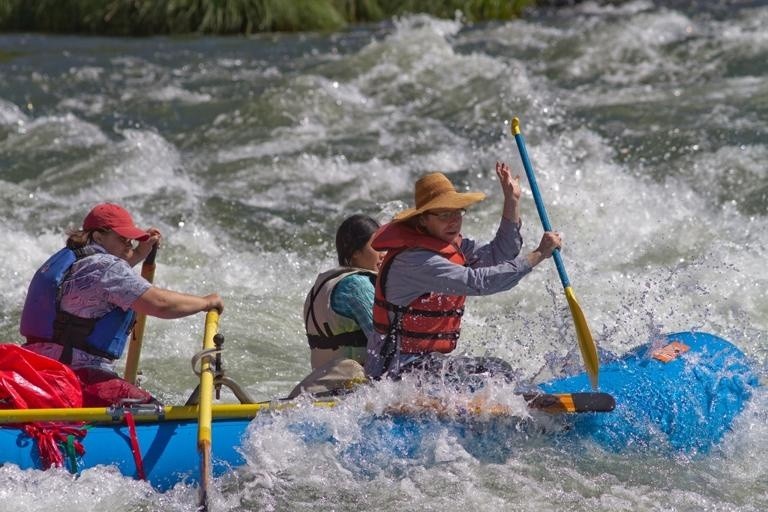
[428,210,467,220]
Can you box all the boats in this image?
[0,333,758,496]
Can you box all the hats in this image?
[83,203,151,242]
[393,172,485,223]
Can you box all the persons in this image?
[18,201,224,409]
[301,213,390,390]
[366,161,564,388]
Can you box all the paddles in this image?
[0,392,616,414]
[510,117,598,391]
[197,307,218,511]
